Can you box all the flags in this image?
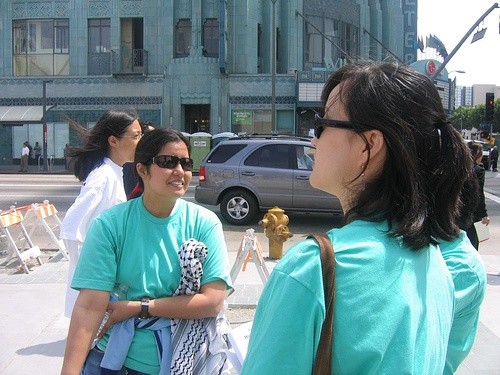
[417,34,447,60]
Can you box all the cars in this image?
[463,138,492,170]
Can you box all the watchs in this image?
[137,297,151,320]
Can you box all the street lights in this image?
[42,79,54,173]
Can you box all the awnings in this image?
[0,105,160,123]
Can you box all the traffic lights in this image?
[480,131,489,140]
[486,93,495,123]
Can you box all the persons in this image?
[58,106,157,317]
[18,141,42,172]
[61,127,235,375]
[240,60,500,375]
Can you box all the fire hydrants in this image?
[259,204,294,260]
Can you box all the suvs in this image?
[194,133,345,226]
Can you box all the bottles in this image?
[90,283,129,350]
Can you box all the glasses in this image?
[314,113,355,139]
[146,155,194,171]
[118,134,144,141]
